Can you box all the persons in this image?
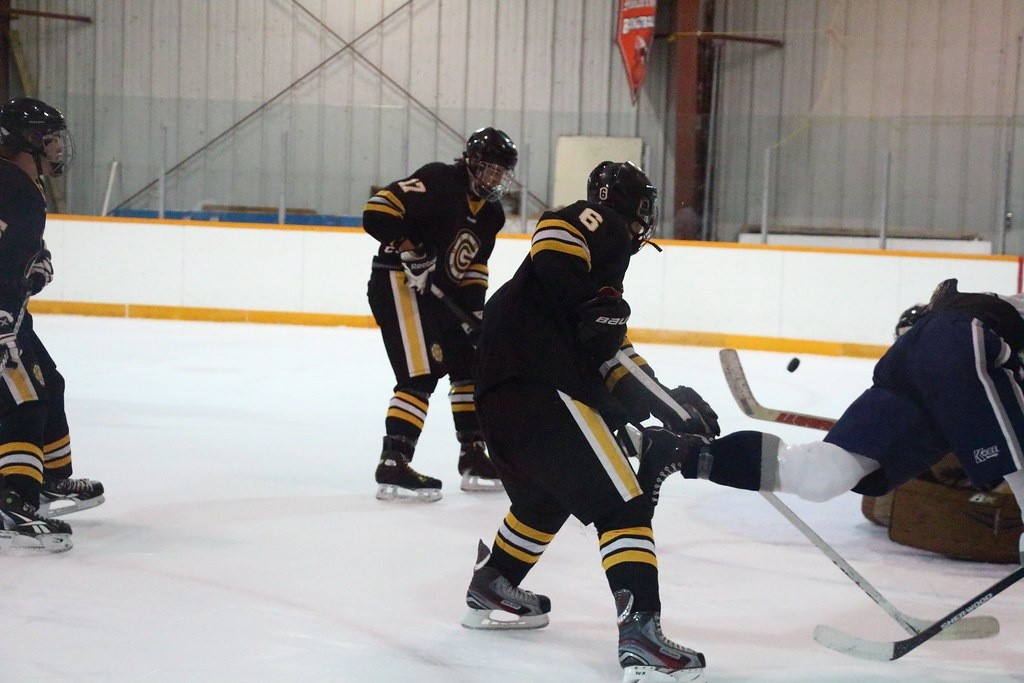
[620,279,1024,568]
[0,96,107,555]
[363,127,518,503]
[459,160,720,683]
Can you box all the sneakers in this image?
[454,430,507,491]
[34,475,106,518]
[636,426,690,510]
[376,436,443,504]
[462,537,551,630]
[613,588,706,682]
[0,472,75,553]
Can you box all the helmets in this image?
[587,159,658,254]
[465,127,519,204]
[1,97,76,178]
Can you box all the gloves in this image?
[398,241,437,296]
[570,296,630,363]
[463,308,485,347]
[19,248,54,296]
[0,309,23,371]
[664,386,721,440]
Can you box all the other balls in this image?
[786,357,800,373]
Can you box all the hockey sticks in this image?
[719,347,840,431]
[430,284,646,462]
[615,349,1001,644]
[811,566,1024,662]
